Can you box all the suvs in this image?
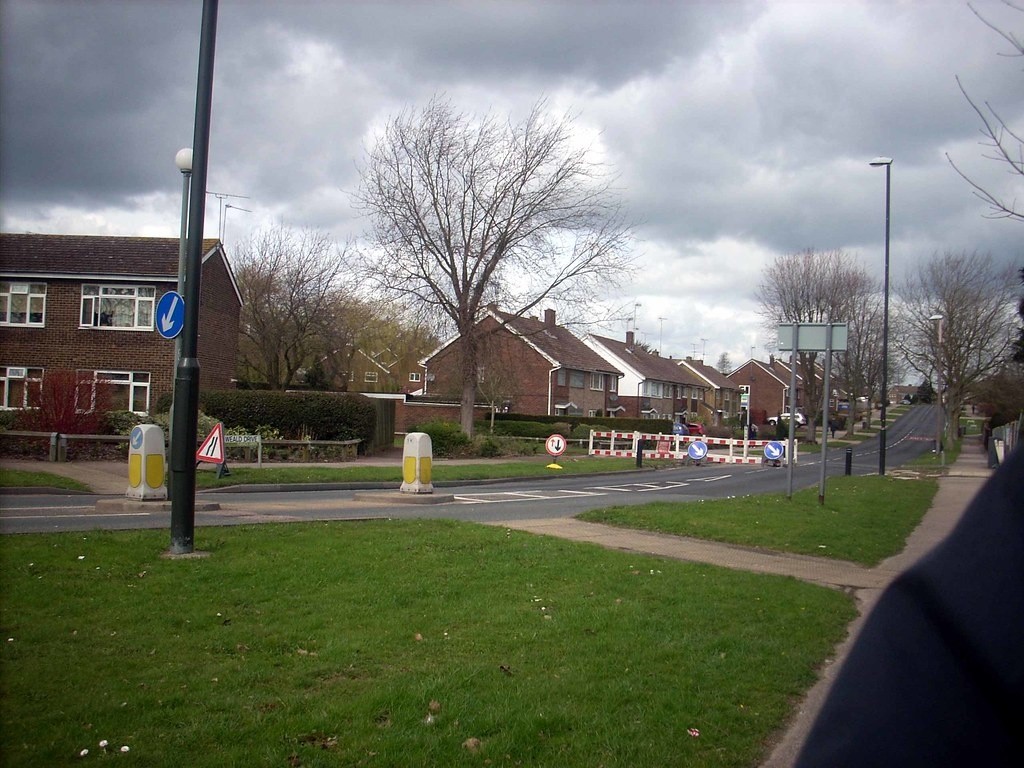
[767,411,807,426]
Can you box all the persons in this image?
[830,418,837,438]
[748,424,758,449]
[680,406,688,424]
[972,404,976,414]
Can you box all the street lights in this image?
[928,312,943,454]
[163,147,193,503]
[869,155,894,475]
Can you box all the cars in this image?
[898,399,911,406]
[684,422,705,437]
[673,422,689,437]
